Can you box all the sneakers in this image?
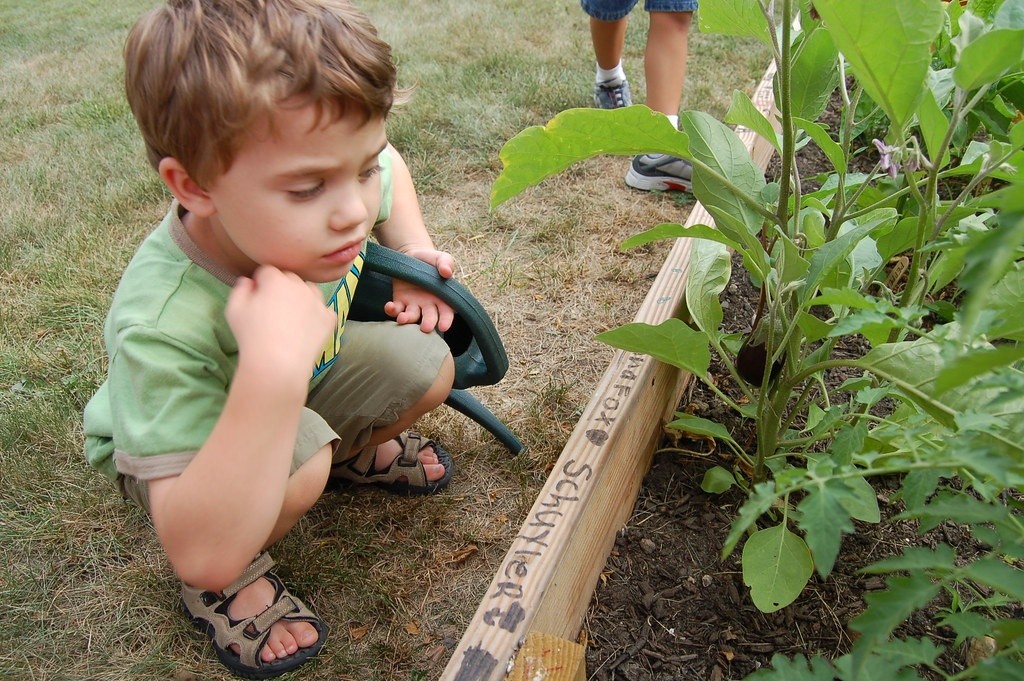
[624,155,694,194]
[594,79,633,110]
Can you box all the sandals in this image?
[325,431,453,494]
[180,550,328,679]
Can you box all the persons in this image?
[84,0,462,678]
[580,0,696,193]
